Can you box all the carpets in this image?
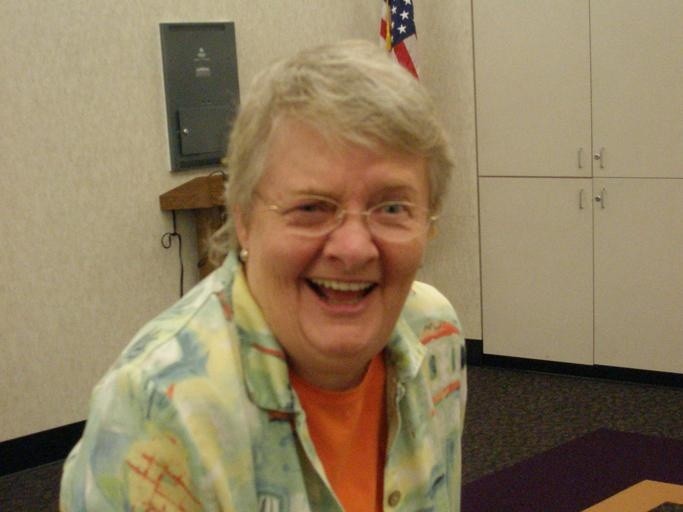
[461,427,683,511]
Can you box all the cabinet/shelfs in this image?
[471,0,683,372]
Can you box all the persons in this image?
[56,36,472,512]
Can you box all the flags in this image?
[377,0,421,81]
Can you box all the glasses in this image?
[252,191,439,245]
